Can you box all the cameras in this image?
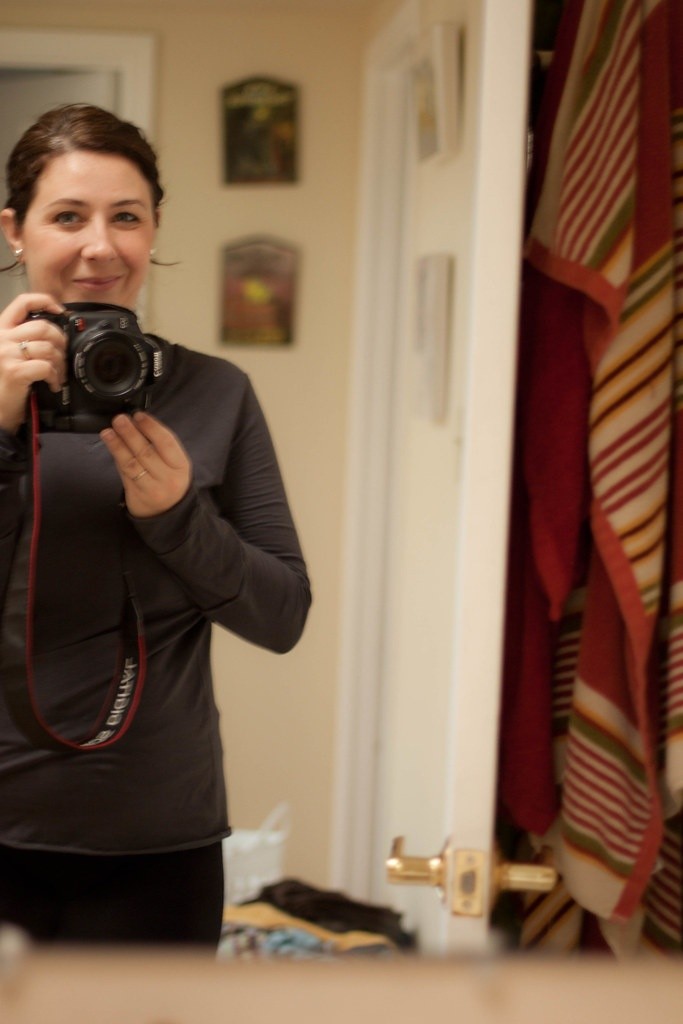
[21,302,170,433]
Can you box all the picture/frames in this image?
[414,254,450,429]
[402,23,460,165]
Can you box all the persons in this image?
[0,104,312,954]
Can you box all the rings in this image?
[19,341,30,360]
[132,469,147,482]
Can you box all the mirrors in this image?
[1,0,683,953]
